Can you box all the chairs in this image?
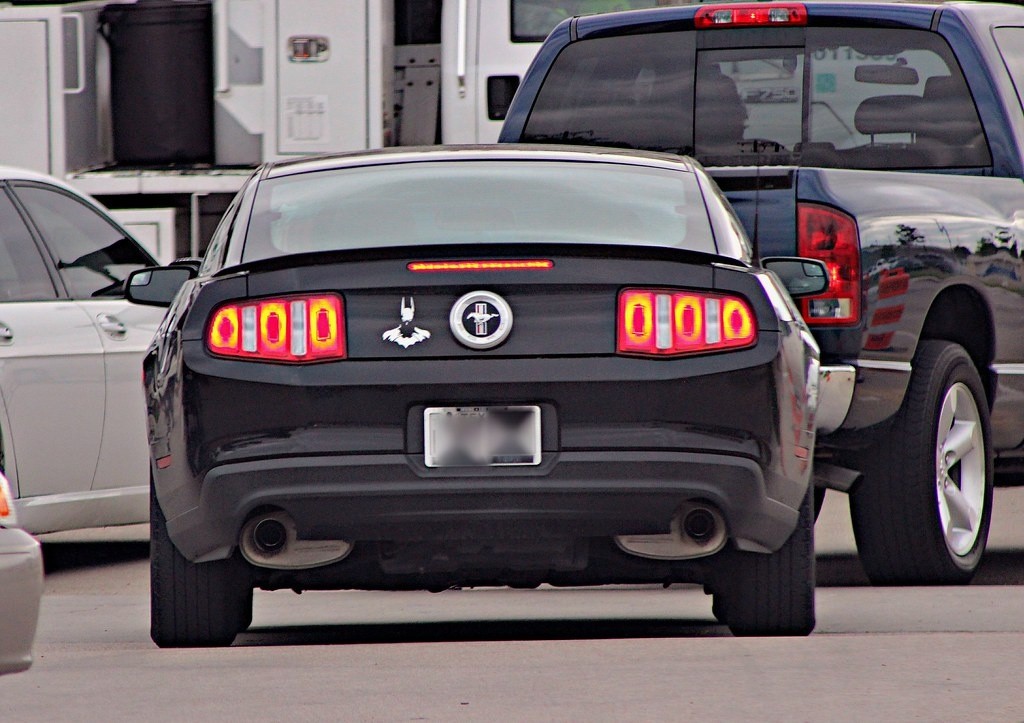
[843,94,933,168]
[915,76,982,145]
[696,73,787,154]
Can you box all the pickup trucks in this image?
[488,1,1024,586]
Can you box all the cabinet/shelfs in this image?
[0,0,137,180]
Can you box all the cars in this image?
[123,142,832,648]
[0,475,44,676]
[0,167,169,534]
